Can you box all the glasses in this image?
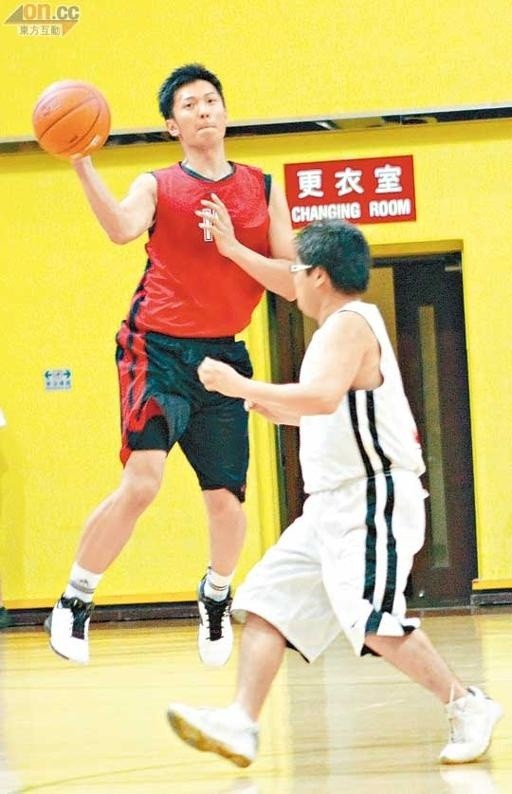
[290,264,312,272]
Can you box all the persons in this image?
[163,214,500,768]
[40,65,301,671]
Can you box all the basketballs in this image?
[31,82,111,162]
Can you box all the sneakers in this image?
[198,573,233,667]
[439,686,503,764]
[44,592,93,663]
[167,704,261,768]
